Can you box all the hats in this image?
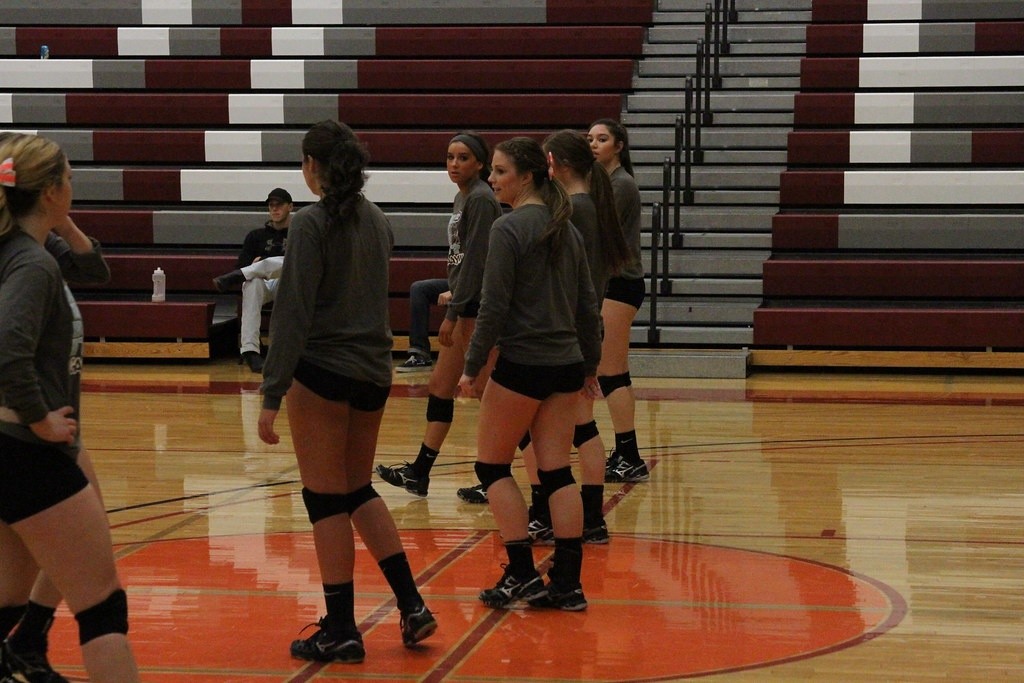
[266,188,292,205]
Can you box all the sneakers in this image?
[399,597,437,646]
[394,351,434,372]
[604,447,651,483]
[289,616,365,663]
[0,638,23,683]
[582,520,608,544]
[6,623,68,683]
[524,581,587,610]
[456,483,489,504]
[375,459,429,497]
[481,564,544,608]
[500,512,555,542]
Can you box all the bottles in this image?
[151,266,166,303]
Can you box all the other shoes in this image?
[213,272,235,293]
[243,352,263,372]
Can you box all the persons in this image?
[461,139,603,610]
[377,133,504,500]
[214,188,293,370]
[396,278,453,371]
[263,120,438,661]
[589,118,649,478]
[503,129,611,544]
[0,213,116,683]
[0,131,142,683]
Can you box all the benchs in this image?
[0,1,660,361]
[756,0,1024,354]
